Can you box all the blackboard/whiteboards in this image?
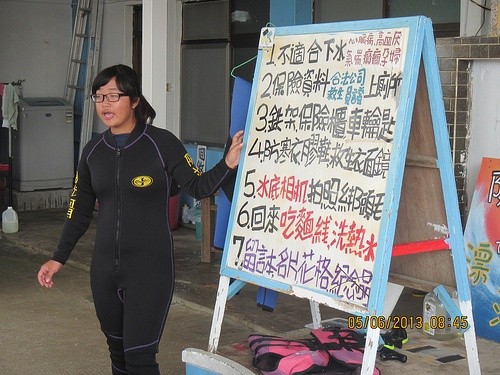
[218,16,475,323]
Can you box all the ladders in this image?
[62,0,105,166]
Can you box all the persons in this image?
[36,64,245,375]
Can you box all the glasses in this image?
[89,93,129,102]
